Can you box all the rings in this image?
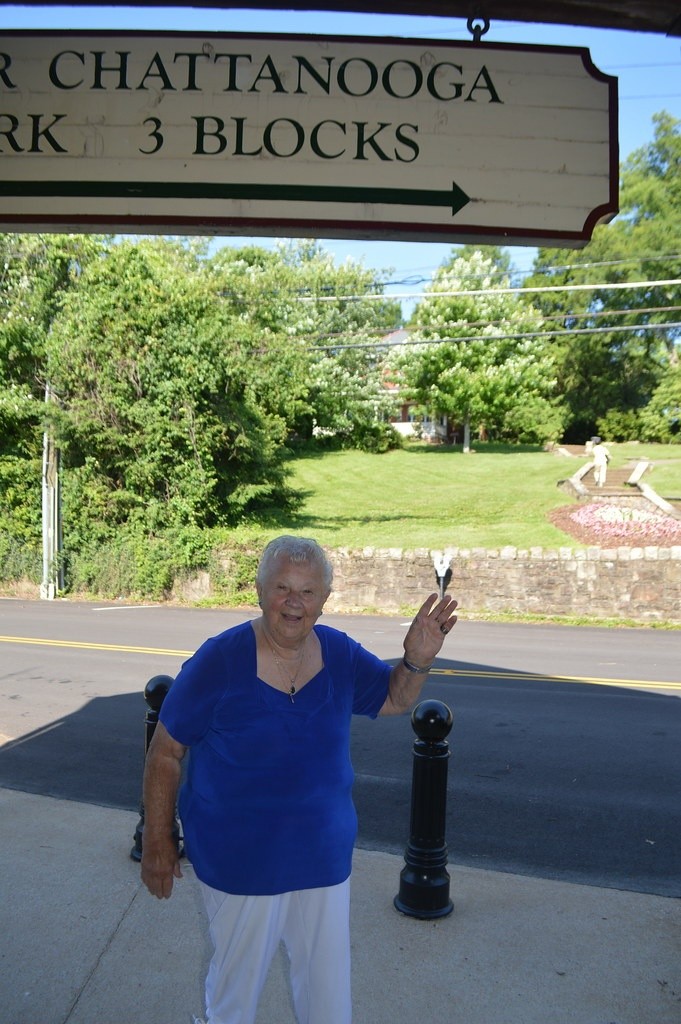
[440,625,449,634]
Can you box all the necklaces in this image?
[270,644,304,703]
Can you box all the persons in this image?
[140,536,458,1024]
[593,438,611,488]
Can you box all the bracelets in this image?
[403,658,436,673]
